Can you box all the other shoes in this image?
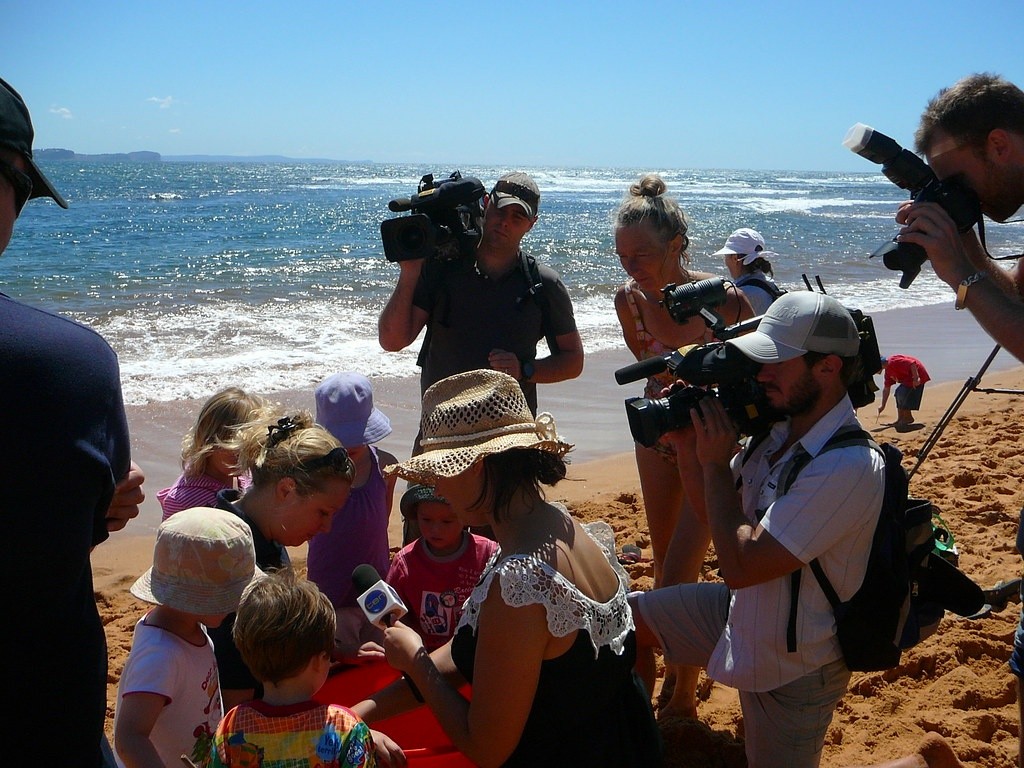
[942,605,992,621]
[981,578,1024,604]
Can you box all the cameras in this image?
[841,122,984,289]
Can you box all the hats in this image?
[878,353,886,363]
[725,290,860,363]
[400,484,436,526]
[382,369,575,484]
[489,171,540,221]
[129,507,270,616]
[315,374,392,447]
[0,78,67,209]
[712,227,779,266]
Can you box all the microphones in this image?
[351,564,427,704]
[389,197,411,212]
[615,355,671,385]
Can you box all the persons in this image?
[205,569,409,768]
[156,388,262,520]
[305,384,397,673]
[0,78,145,768]
[876,354,930,426]
[661,293,960,768]
[895,75,1024,768]
[349,369,664,768]
[615,179,757,719]
[377,175,584,546]
[207,409,356,709]
[713,228,778,316]
[113,507,269,768]
[386,484,497,653]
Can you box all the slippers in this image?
[615,551,650,565]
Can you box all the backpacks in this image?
[733,423,983,673]
[929,514,959,569]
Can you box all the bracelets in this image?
[955,270,988,310]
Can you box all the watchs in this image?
[519,357,535,384]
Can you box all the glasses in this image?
[275,449,348,475]
[0,158,33,219]
